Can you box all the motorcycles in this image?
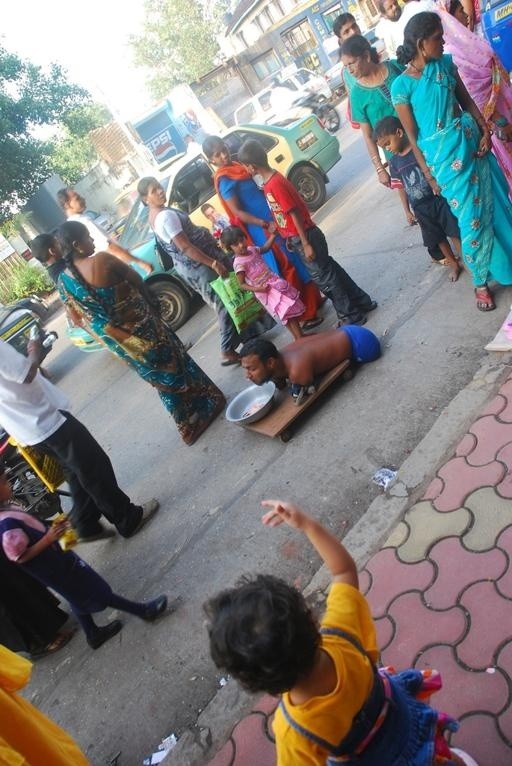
[0,431,66,529]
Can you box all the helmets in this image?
[270,78,280,88]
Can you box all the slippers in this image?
[30,631,70,660]
[475,286,496,311]
[432,256,459,265]
[220,353,242,366]
[333,316,368,329]
[360,299,378,315]
[298,316,324,329]
[315,292,328,311]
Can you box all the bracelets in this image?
[211,260,218,270]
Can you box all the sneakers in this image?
[129,498,160,537]
[78,527,116,542]
[449,748,480,766]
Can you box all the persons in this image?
[183,134,202,154]
[1,334,160,543]
[218,223,306,340]
[26,173,234,448]
[1,559,75,655]
[237,324,380,406]
[201,498,478,766]
[235,137,378,330]
[261,0,511,311]
[200,202,230,240]
[200,134,328,330]
[1,463,172,655]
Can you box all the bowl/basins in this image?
[226,380,276,426]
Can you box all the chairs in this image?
[193,174,216,204]
[173,188,193,212]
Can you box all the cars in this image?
[78,205,115,233]
[229,26,399,151]
[0,281,49,356]
[66,116,343,359]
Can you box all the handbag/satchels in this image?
[154,241,174,272]
[8,434,68,493]
[209,271,267,336]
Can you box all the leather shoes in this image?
[87,619,123,650]
[144,594,167,622]
